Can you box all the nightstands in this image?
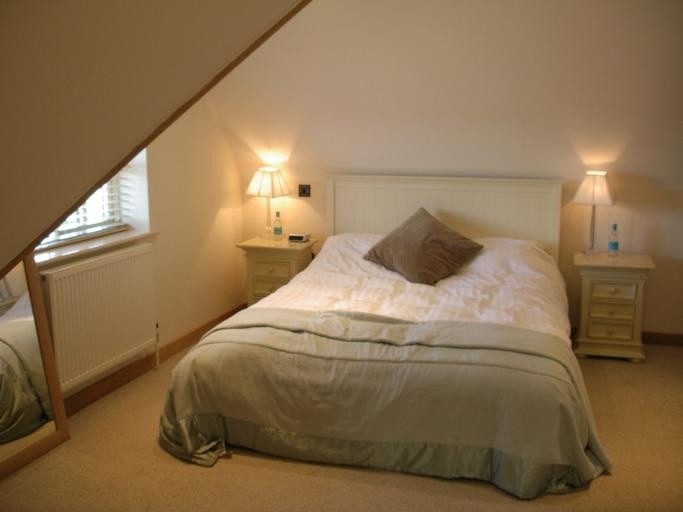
[234,232,319,307]
[570,250,656,364]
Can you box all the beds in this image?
[155,174,595,482]
[0,292,54,444]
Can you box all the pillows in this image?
[361,206,484,288]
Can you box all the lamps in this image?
[571,169,612,257]
[244,166,292,239]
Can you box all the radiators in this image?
[39,240,158,397]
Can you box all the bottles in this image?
[273,211,284,241]
[607,223,619,258]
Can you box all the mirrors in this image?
[0,253,72,483]
